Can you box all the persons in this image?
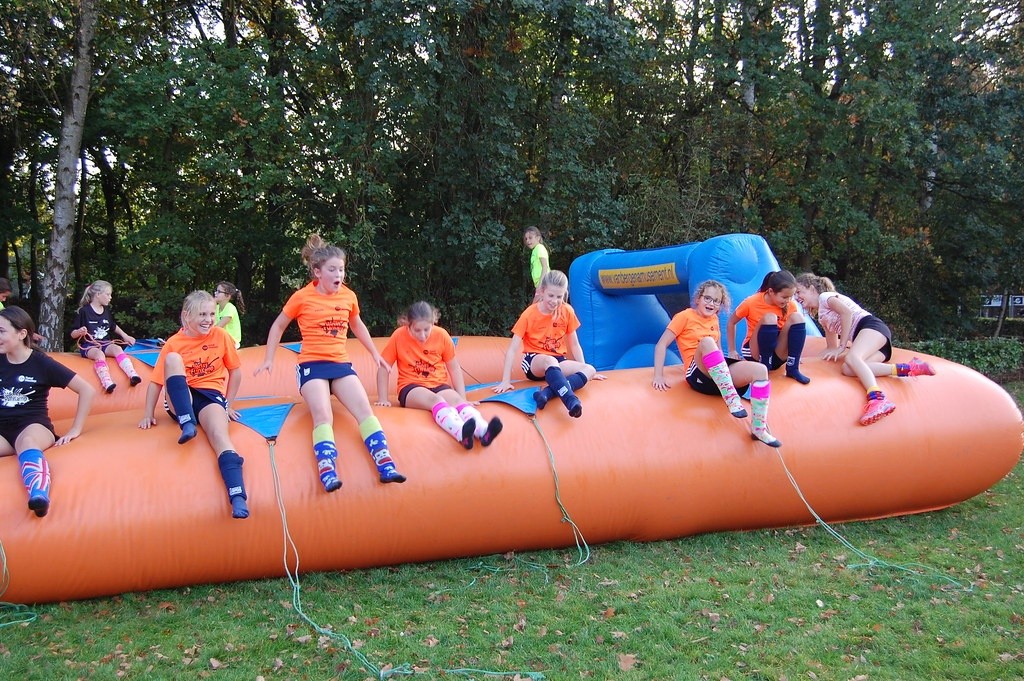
[652,280,782,447]
[70,281,141,393]
[523,227,551,288]
[0,278,44,340]
[214,282,245,349]
[139,291,249,518]
[254,235,407,493]
[793,273,938,424]
[727,269,810,384]
[374,301,503,449]
[0,306,95,518]
[492,270,608,418]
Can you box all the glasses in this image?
[701,294,722,306]
[215,289,229,294]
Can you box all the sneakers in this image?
[859,397,896,426]
[908,357,936,381]
